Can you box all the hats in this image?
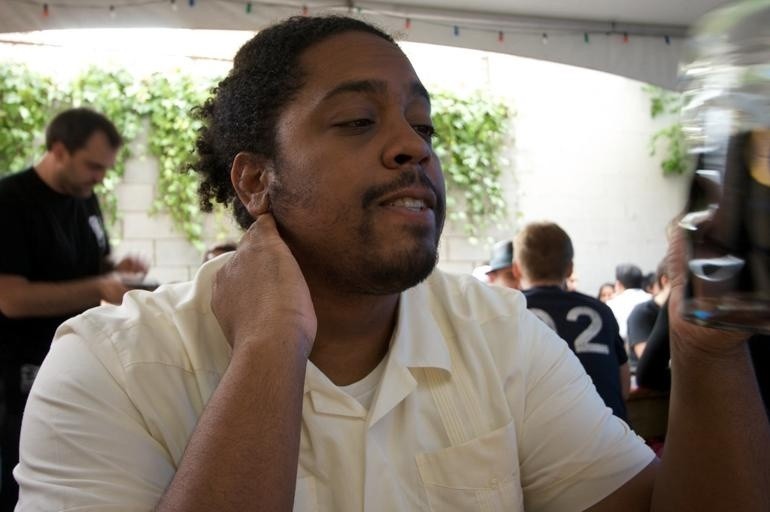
[486,239,513,274]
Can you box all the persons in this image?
[13,13,769,512]
[488,220,632,422]
[201,242,237,262]
[0,107,149,512]
[599,258,768,409]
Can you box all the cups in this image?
[676,13,768,330]
